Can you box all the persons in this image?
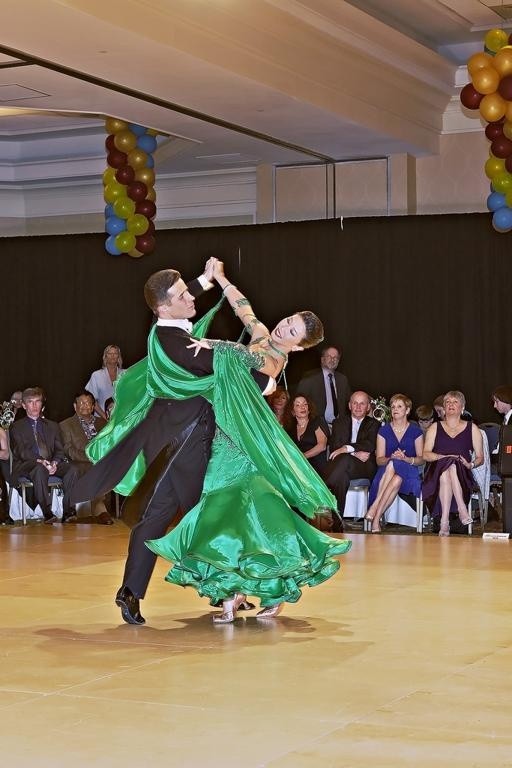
[269,383,483,536]
[3,390,120,529]
[112,255,255,629]
[292,345,353,439]
[81,345,129,418]
[184,256,326,623]
[490,386,511,536]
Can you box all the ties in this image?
[35,421,48,460]
[328,373,338,418]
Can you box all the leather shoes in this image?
[116,586,145,625]
[332,514,387,536]
[436,515,474,535]
[210,599,255,610]
[1,509,114,526]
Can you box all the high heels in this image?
[257,604,284,616]
[213,592,250,623]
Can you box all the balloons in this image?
[456,24,512,236]
[97,111,160,267]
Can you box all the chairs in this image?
[6,411,504,535]
